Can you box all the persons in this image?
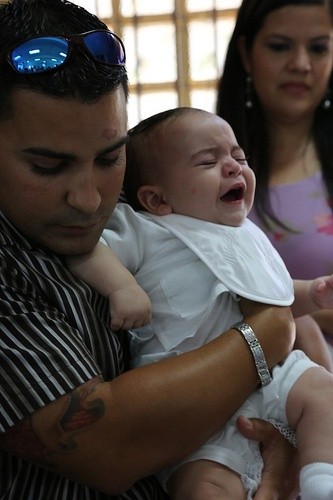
[214,0,332,371]
[0,1,295,500]
[57,105,332,500]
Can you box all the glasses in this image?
[1,29,127,75]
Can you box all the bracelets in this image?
[234,321,273,388]
